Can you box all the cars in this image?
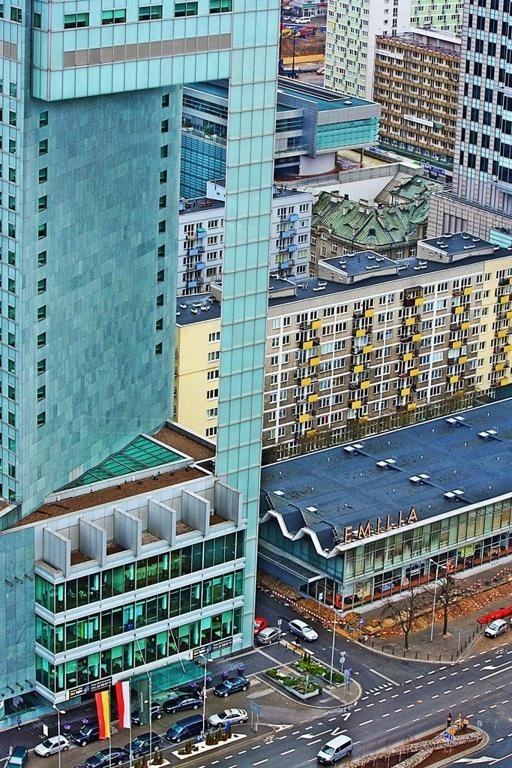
[6,676,250,768]
[254,617,282,644]
[282,16,311,24]
[484,617,512,638]
[288,619,318,641]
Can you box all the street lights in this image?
[329,605,343,686]
[430,559,447,642]
[292,29,296,76]
[199,654,213,734]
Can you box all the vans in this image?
[317,735,352,765]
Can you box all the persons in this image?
[446,711,452,728]
[16,714,25,731]
[461,716,470,732]
[455,713,463,731]
